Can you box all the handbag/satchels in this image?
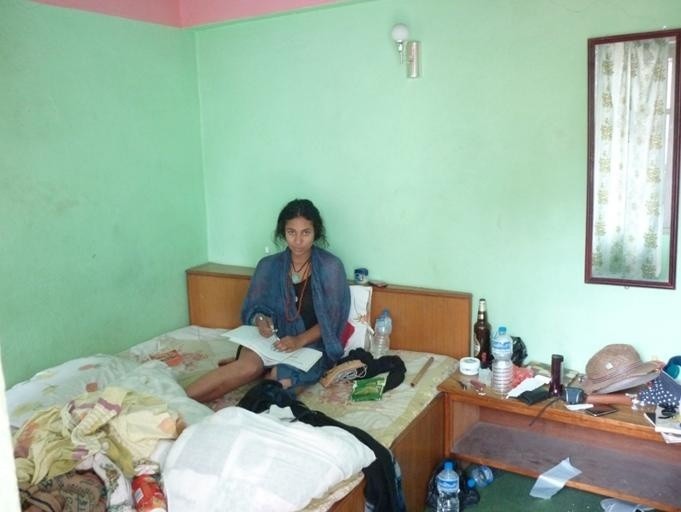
[638,355,681,409]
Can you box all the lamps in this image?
[391,24,419,78]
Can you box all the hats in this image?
[573,343,664,395]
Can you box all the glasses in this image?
[658,403,676,419]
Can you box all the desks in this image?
[436,367,681,512]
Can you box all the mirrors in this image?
[584,29,681,289]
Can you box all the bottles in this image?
[371,308,393,359]
[434,461,461,512]
[473,298,492,369]
[490,325,514,395]
[467,463,505,490]
[131,474,168,512]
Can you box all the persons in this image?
[185,197,353,404]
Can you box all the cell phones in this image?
[585,404,617,416]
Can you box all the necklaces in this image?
[291,255,311,284]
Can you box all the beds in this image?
[4,263,469,512]
